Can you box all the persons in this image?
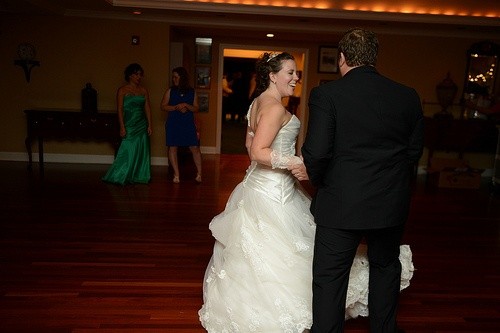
[101,63,152,184]
[287,28,423,333]
[198,52,414,333]
[465,98,500,193]
[161,66,202,182]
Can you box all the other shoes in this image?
[173,175,179,184]
[195,173,201,183]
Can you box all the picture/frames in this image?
[195,44,212,64]
[194,66,211,89]
[318,46,339,74]
[197,92,209,112]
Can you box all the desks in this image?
[24,108,121,170]
[422,117,500,182]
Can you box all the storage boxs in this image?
[424,156,486,190]
